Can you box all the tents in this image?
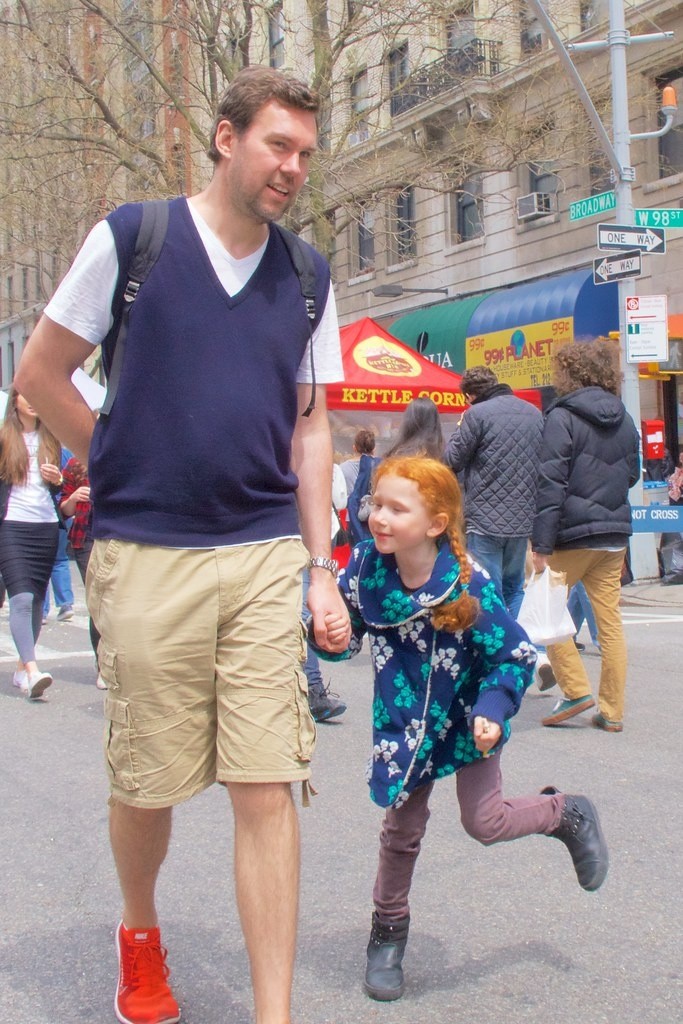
[322,315,470,415]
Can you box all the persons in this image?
[306,454,611,1000]
[442,367,558,691]
[531,342,639,732]
[643,448,676,484]
[0,387,109,697]
[11,66,350,1024]
[520,542,603,654]
[385,398,448,463]
[299,429,382,721]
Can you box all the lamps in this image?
[370,283,449,298]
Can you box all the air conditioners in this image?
[516,191,555,219]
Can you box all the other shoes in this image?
[41,615,48,624]
[571,642,585,652]
[56,604,74,620]
[533,654,557,692]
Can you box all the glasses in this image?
[463,396,471,405]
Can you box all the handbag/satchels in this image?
[515,565,577,647]
[335,517,347,546]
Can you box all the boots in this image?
[540,785,609,892]
[365,911,410,1001]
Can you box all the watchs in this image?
[308,558,339,578]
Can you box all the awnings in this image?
[379,269,620,390]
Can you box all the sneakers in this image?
[115,918,181,1024]
[97,672,107,690]
[542,692,595,726]
[13,671,29,689]
[28,671,53,698]
[308,676,347,722]
[591,713,623,733]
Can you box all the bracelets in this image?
[57,475,64,486]
